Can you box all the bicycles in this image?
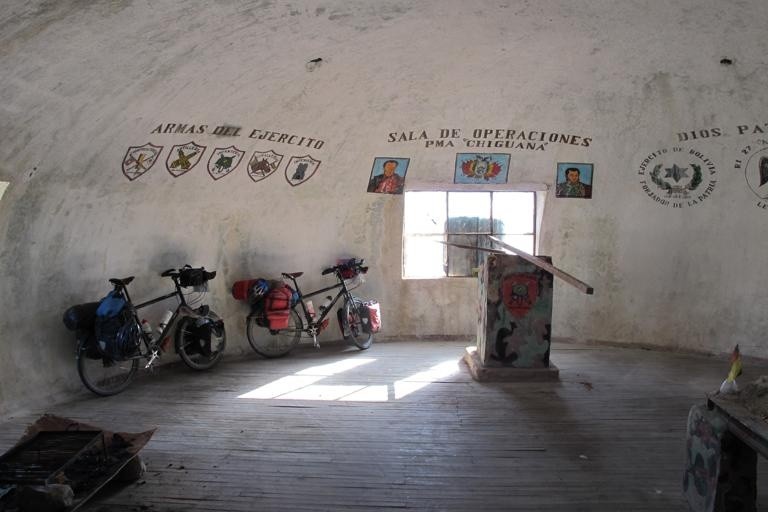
[74,264,226,397]
[245,258,373,360]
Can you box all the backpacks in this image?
[91,287,142,362]
[257,282,292,333]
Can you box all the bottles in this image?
[318,295,333,313]
[141,319,156,344]
[155,308,174,335]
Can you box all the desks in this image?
[683,376,768,512]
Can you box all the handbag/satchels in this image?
[336,308,360,340]
[337,260,359,280]
[360,299,382,334]
[196,319,226,357]
[178,266,208,288]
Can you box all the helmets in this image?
[248,278,270,304]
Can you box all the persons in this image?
[370,160,401,194]
[559,168,586,197]
